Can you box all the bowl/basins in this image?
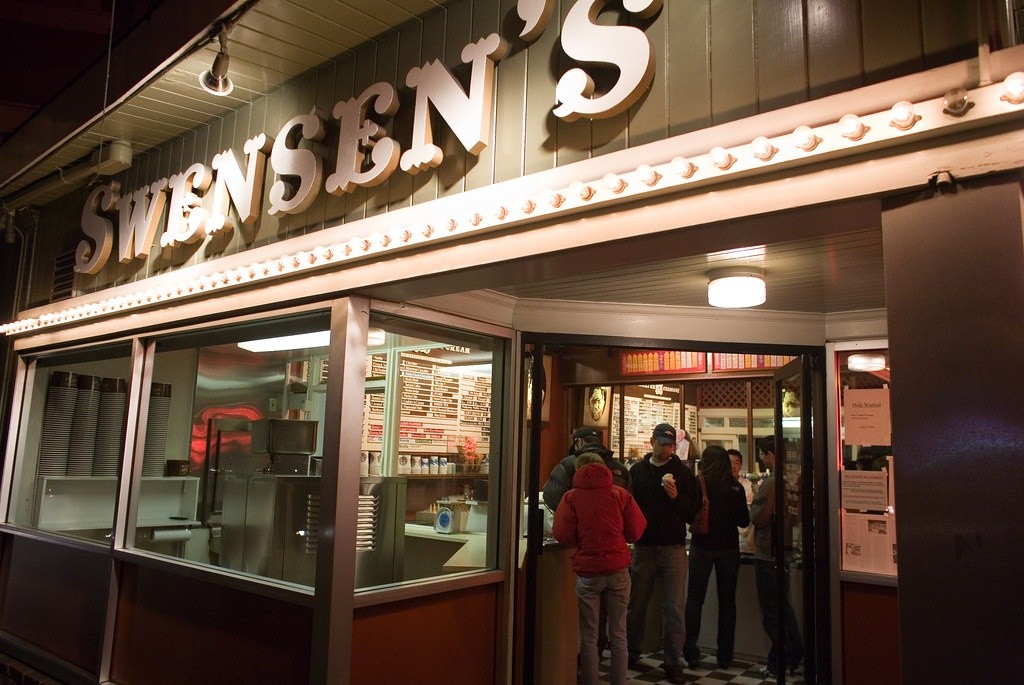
[660,477,676,487]
[166,460,191,476]
[141,382,172,478]
[38,370,126,476]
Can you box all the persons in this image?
[543,427,632,663]
[627,423,698,684]
[844,447,888,515]
[872,522,880,532]
[553,453,647,685]
[727,449,754,533]
[676,429,689,460]
[749,435,800,677]
[682,445,750,670]
[847,544,855,555]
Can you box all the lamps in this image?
[708,265,766,308]
[199,22,233,97]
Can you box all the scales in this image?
[434,499,462,535]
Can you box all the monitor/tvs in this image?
[268,419,319,454]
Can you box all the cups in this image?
[360,451,447,477]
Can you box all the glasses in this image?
[759,453,764,460]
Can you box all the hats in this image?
[654,423,676,444]
[569,426,598,455]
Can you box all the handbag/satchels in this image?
[688,470,709,534]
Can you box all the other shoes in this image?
[761,668,778,678]
[682,646,700,662]
[717,657,729,669]
[665,664,687,684]
[627,656,635,668]
